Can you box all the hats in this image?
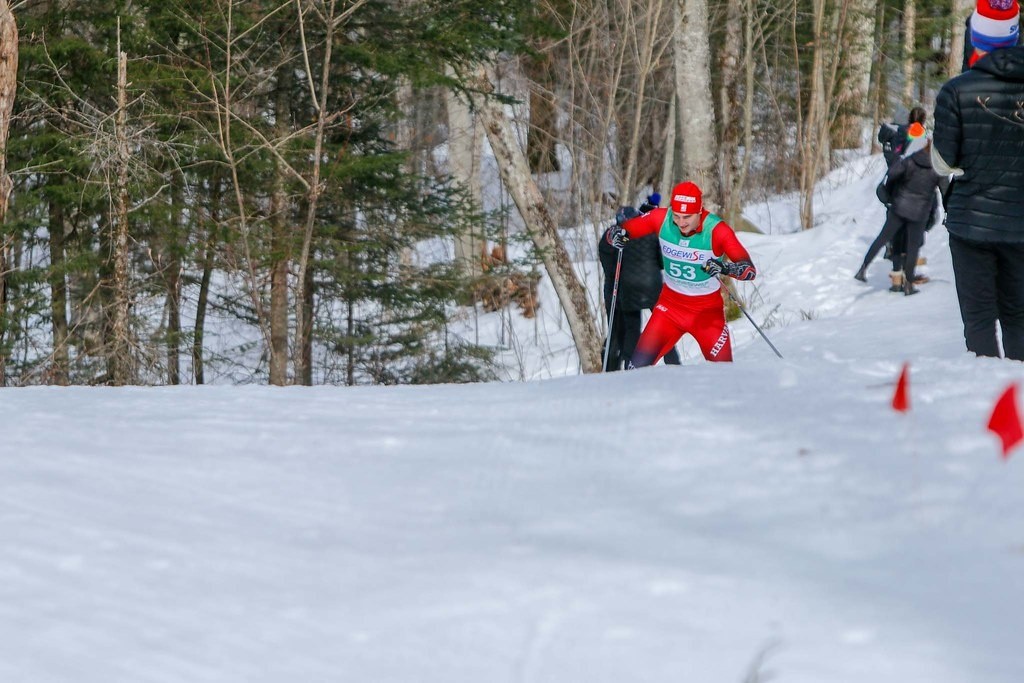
[638,193,660,216]
[616,206,639,224]
[907,122,926,141]
[670,182,702,214]
[970,0,1020,52]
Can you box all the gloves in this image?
[705,258,728,276]
[609,225,630,249]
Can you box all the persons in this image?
[931,0,1024,360]
[606,181,756,370]
[598,194,681,372]
[855,107,937,296]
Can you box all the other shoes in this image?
[912,276,929,284]
[914,257,926,265]
[884,242,893,259]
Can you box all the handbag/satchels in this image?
[877,155,909,203]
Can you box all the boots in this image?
[904,280,919,296]
[889,271,903,291]
[855,264,867,283]
